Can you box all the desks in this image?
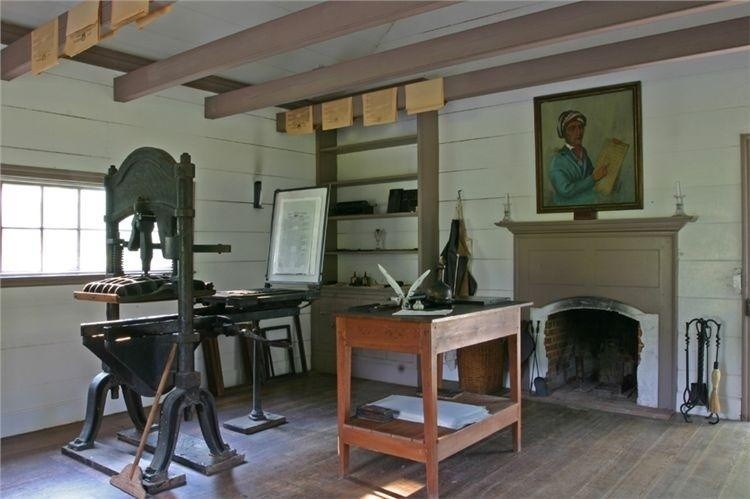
[332,299,536,499]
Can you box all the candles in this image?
[505,193,509,211]
[676,181,682,203]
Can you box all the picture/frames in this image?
[264,182,333,286]
[532,80,645,214]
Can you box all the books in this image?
[589,138,630,196]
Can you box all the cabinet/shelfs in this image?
[310,110,440,388]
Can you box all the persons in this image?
[547,110,621,206]
